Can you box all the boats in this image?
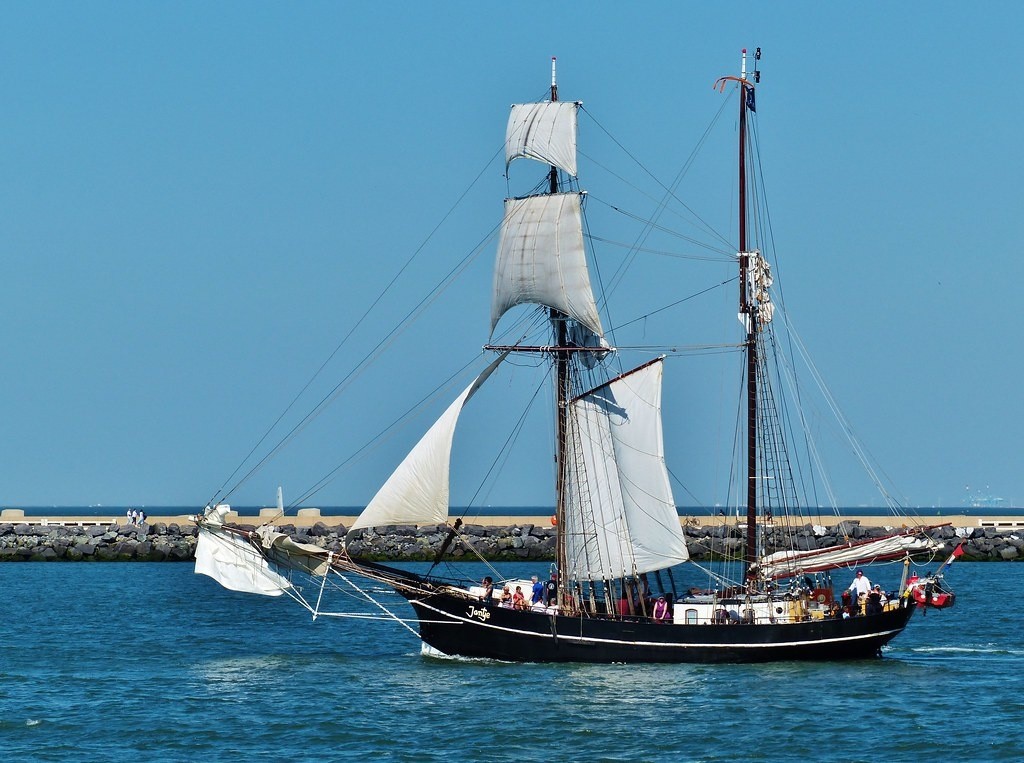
[906,569,957,612]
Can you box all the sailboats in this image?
[188,44,964,668]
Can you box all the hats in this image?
[552,574,557,580]
[658,595,665,599]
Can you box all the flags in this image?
[944,542,964,570]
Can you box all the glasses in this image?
[857,573,862,575]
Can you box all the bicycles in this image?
[683,513,701,527]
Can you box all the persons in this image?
[618,592,675,620]
[480,573,587,615]
[934,578,941,593]
[821,569,888,619]
